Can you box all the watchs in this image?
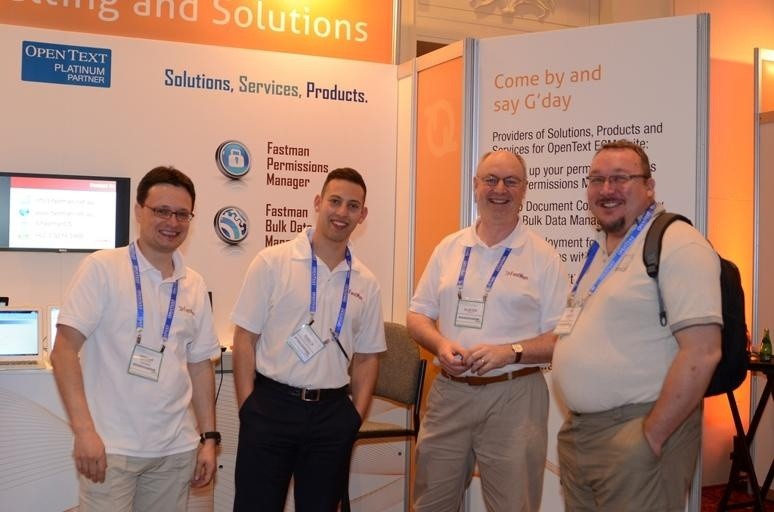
[200,431,222,444]
[511,342,524,363]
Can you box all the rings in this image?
[481,358,486,365]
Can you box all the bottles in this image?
[760,329,771,362]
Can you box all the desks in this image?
[717,355,774,512]
[0,353,239,512]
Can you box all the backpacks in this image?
[644,210,747,395]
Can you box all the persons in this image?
[409,150,567,512]
[51,167,220,512]
[553,140,723,512]
[233,167,387,512]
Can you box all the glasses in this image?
[585,173,652,185]
[143,203,196,225]
[474,175,524,190]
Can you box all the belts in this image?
[256,371,352,405]
[439,367,543,387]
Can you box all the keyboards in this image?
[0,358,46,370]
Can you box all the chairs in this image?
[356,321,428,442]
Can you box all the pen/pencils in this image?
[329,328,350,362]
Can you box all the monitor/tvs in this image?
[47,305,78,363]
[0,171,130,252]
[0,307,42,362]
